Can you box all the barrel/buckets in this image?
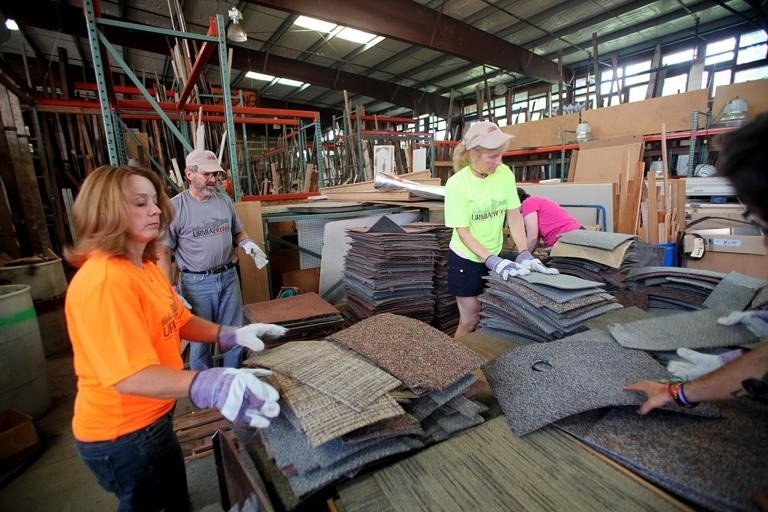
[660,243,675,266]
[0,284,51,416]
[0,259,71,358]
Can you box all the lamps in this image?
[226,20,248,42]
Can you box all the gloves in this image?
[514,252,559,276]
[215,322,288,354]
[239,237,268,270]
[484,254,531,282]
[187,366,280,430]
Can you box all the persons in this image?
[620,110,766,416]
[444,120,561,338]
[156,148,272,373]
[516,188,585,260]
[59,166,290,511]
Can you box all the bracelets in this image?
[669,382,700,408]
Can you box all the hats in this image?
[465,121,514,150]
[186,150,225,174]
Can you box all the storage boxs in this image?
[0,408,40,480]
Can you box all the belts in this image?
[180,261,237,275]
